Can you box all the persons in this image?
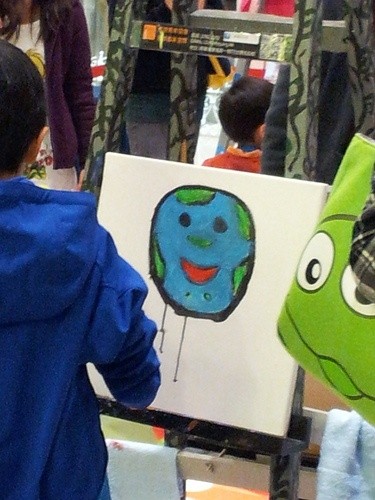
[0,37,161,500]
[0,1,97,193]
[202,77,275,173]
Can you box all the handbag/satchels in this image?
[278,133,374,429]
